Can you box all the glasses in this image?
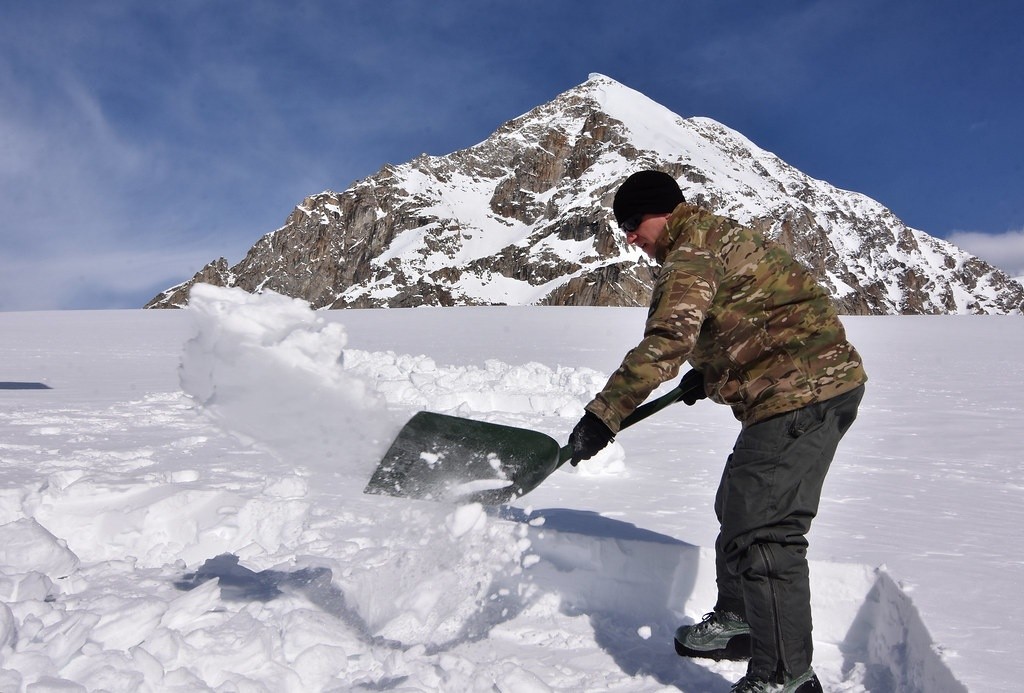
[620,214,643,232]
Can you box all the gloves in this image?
[568,411,612,466]
[674,367,708,405]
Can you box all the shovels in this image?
[361,383,696,506]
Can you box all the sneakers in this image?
[729,665,824,693]
[674,612,752,662]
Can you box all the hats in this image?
[613,170,686,227]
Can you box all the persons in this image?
[568,170,868,693]
